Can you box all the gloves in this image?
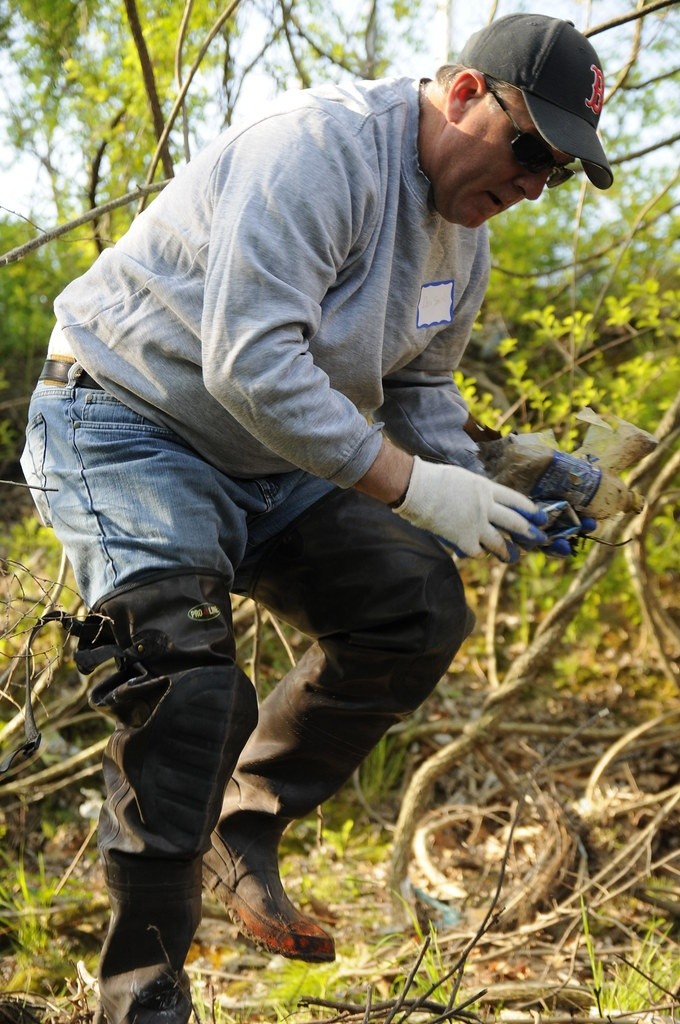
[390,454,596,565]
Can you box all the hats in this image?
[457,11,615,190]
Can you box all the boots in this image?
[0,566,261,1023]
[201,486,468,964]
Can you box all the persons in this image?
[21,14,615,1024]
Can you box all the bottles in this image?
[486,434,646,520]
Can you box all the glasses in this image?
[484,81,576,189]
